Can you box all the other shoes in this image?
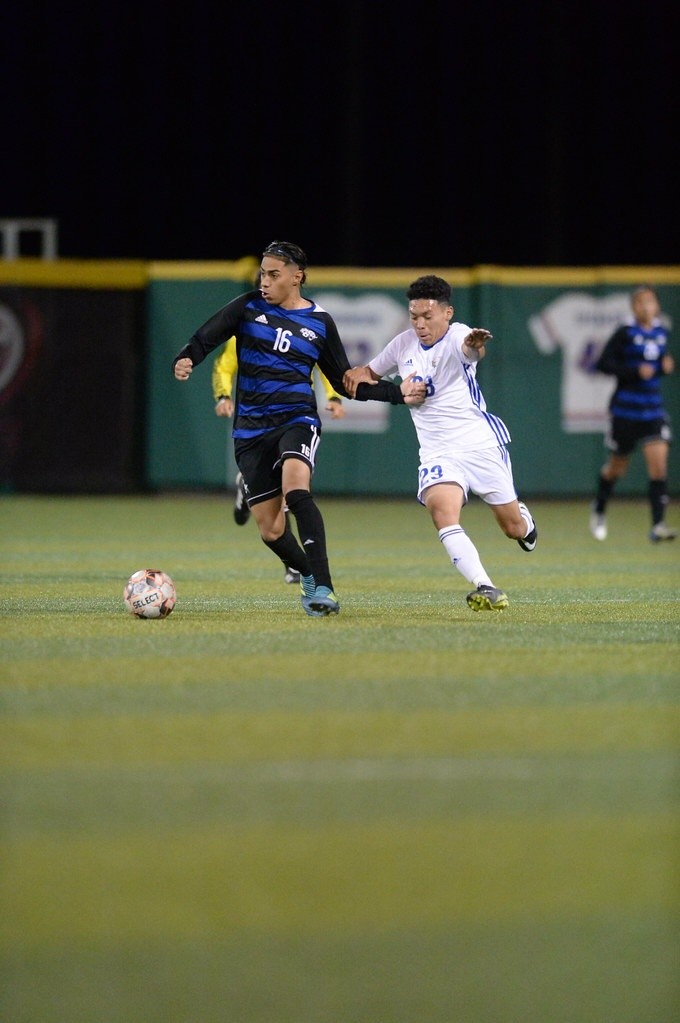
[589,499,608,540]
[648,524,678,541]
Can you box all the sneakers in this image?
[283,568,302,586]
[308,585,340,614]
[517,500,538,553]
[465,584,509,612]
[299,574,319,616]
[233,471,251,526]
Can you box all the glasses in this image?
[265,241,292,259]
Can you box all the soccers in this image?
[124,569,176,619]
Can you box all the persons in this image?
[589,285,677,543]
[170,240,425,617]
[341,272,538,611]
